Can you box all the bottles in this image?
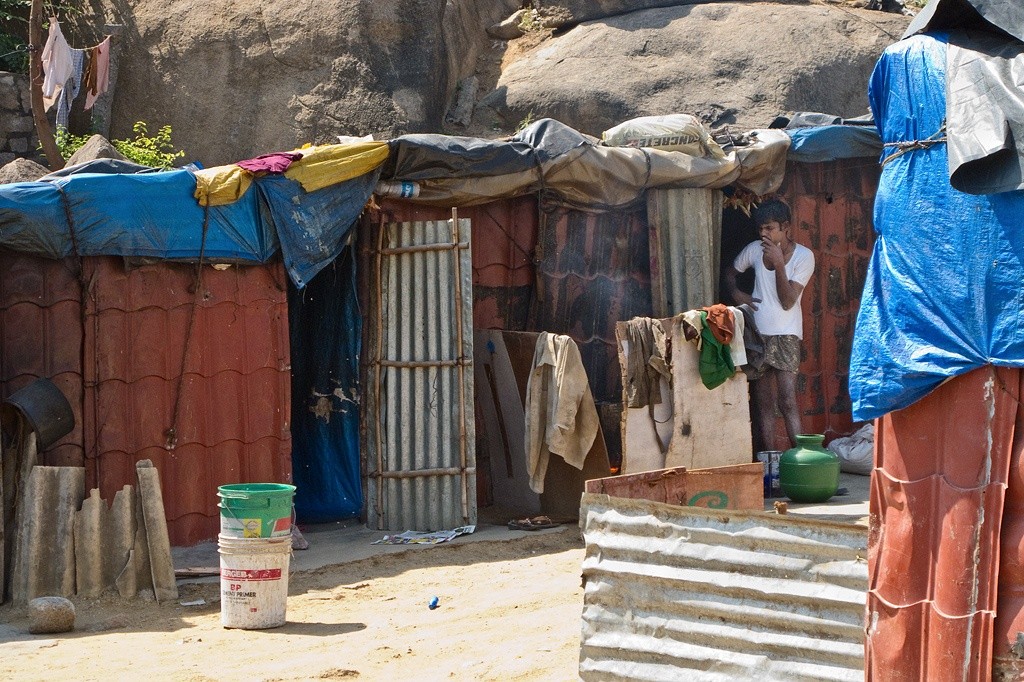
[374,179,420,200]
[779,433,841,503]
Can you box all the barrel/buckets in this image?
[217,533,297,630]
[0,378,75,454]
[757,450,784,499]
[216,482,297,538]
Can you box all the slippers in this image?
[531,516,560,528]
[508,519,540,531]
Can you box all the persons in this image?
[722,198,815,451]
